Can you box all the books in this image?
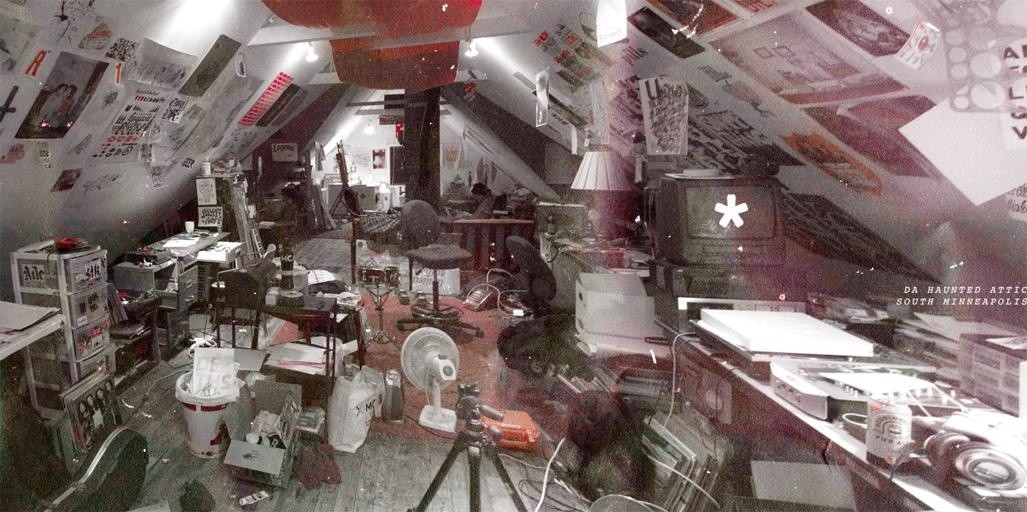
[899,312,1021,382]
[547,357,734,512]
[108,282,128,326]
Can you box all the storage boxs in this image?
[959,332,1027,419]
[575,271,663,337]
[221,379,303,489]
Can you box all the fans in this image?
[401,326,460,431]
[400,200,440,292]
[505,234,555,310]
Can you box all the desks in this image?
[654,311,1026,512]
[262,292,337,380]
[146,228,232,258]
[1,312,65,362]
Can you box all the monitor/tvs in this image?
[655,177,787,269]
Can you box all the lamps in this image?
[571,150,632,236]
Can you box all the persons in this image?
[24,265,31,281]
[86,263,102,277]
[789,58,833,84]
[39,270,44,279]
[30,266,38,280]
[76,382,121,448]
[465,181,494,218]
[39,84,77,121]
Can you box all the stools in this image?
[406,243,483,336]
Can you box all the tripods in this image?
[415,382,527,512]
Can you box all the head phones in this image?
[889,432,1027,490]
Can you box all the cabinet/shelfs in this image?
[10,242,114,417]
[111,301,160,395]
[115,258,199,350]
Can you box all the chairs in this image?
[208,243,276,348]
